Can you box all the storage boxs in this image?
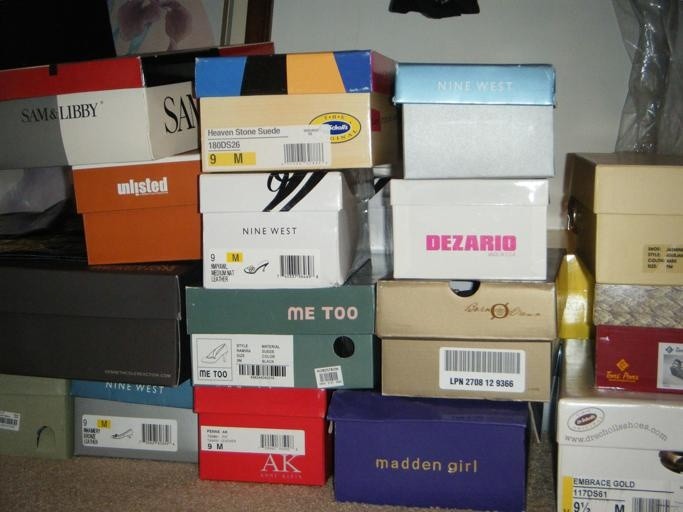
[0,262,186,387]
[555,371,683,512]
[327,390,529,512]
[374,282,563,400]
[66,380,199,463]
[591,286,683,394]
[69,159,200,265]
[195,47,397,173]
[565,150,683,284]
[200,171,371,290]
[391,180,549,281]
[0,373,72,461]
[193,390,326,486]
[1,41,275,169]
[395,61,558,179]
[186,254,391,389]
